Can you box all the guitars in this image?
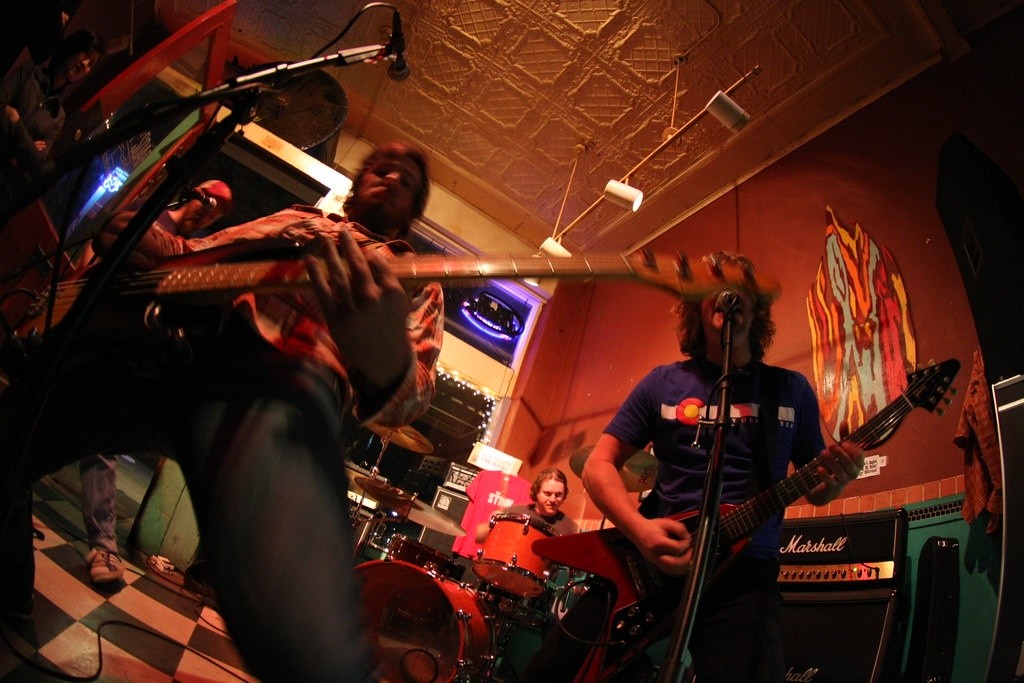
[530,356,964,683]
[14,245,760,340]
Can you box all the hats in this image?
[195,178,232,216]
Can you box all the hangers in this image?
[503,454,518,477]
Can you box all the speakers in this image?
[418,486,481,587]
[350,506,375,557]
[691,585,907,683]
[980,370,1024,683]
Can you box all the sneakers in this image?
[88,546,124,583]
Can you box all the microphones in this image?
[185,186,217,211]
[718,290,739,312]
[387,12,409,81]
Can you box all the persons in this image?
[80,179,238,580]
[1,136,441,682]
[0,27,104,221]
[475,469,579,599]
[581,251,864,682]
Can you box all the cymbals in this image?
[351,404,434,455]
[568,443,660,491]
[354,476,467,536]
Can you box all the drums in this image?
[355,558,495,683]
[472,512,558,601]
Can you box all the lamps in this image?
[600,174,643,214]
[708,87,753,132]
[540,235,571,268]
[523,250,546,286]
